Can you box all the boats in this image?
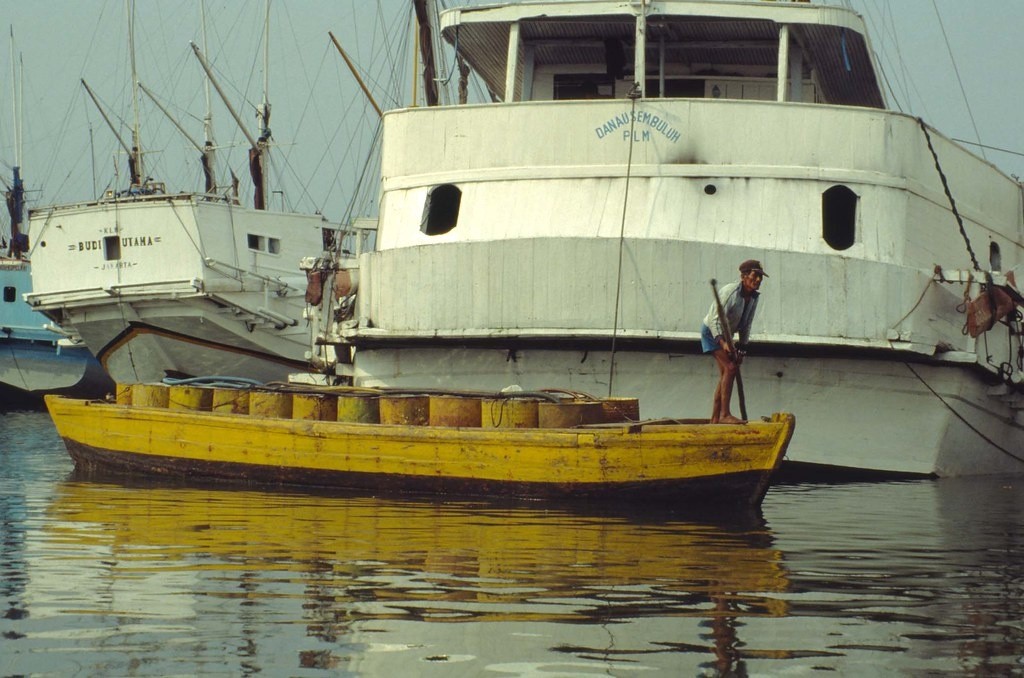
[23,2,359,389]
[48,382,797,510]
[293,2,1024,483]
[0,23,117,394]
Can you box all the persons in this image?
[700,259,769,423]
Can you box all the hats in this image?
[739,260,769,278]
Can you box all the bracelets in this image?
[738,350,747,356]
